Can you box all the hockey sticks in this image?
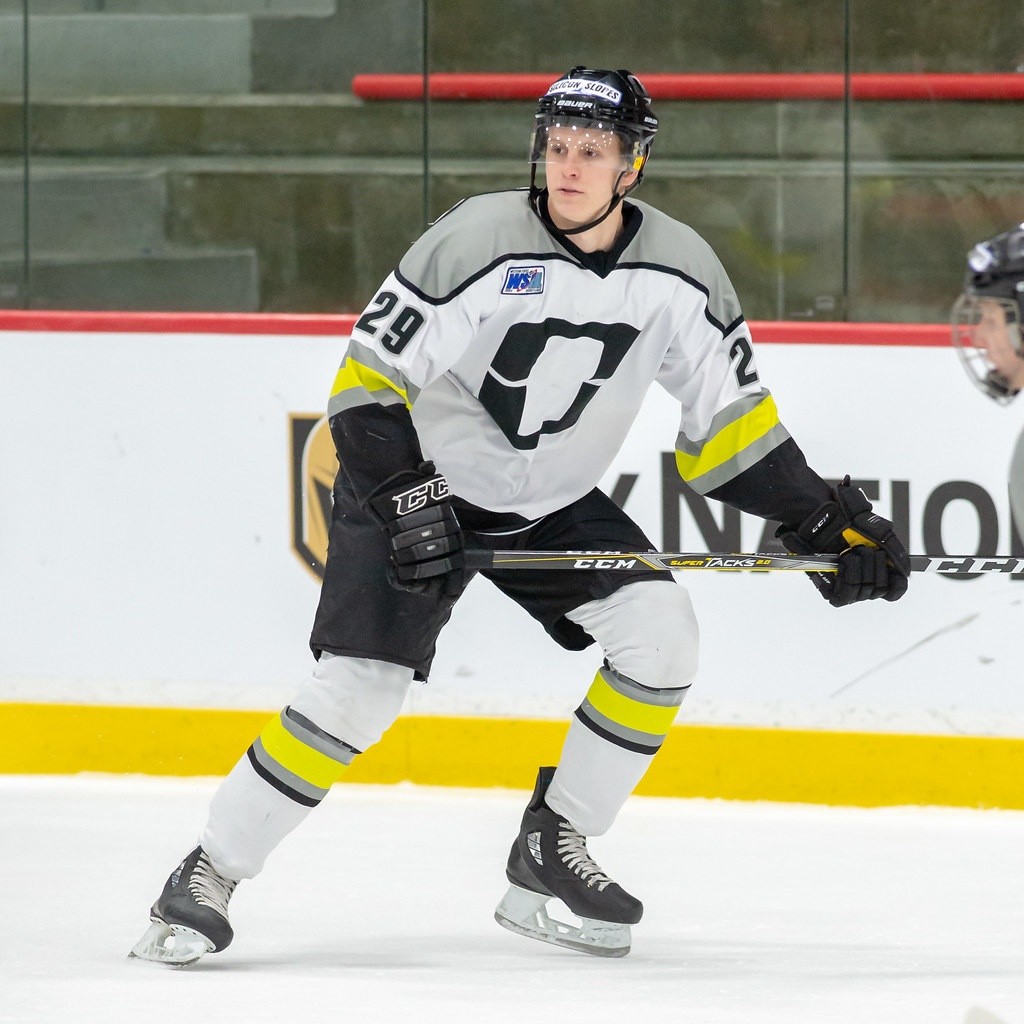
[449,551,1023,574]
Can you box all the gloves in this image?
[776,475,911,607]
[363,459,469,613]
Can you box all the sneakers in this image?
[129,845,239,969]
[496,766,643,959]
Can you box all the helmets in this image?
[531,65,660,171]
[949,223,1024,405]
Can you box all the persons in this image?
[127,64,911,971]
[951,222,1024,552]
